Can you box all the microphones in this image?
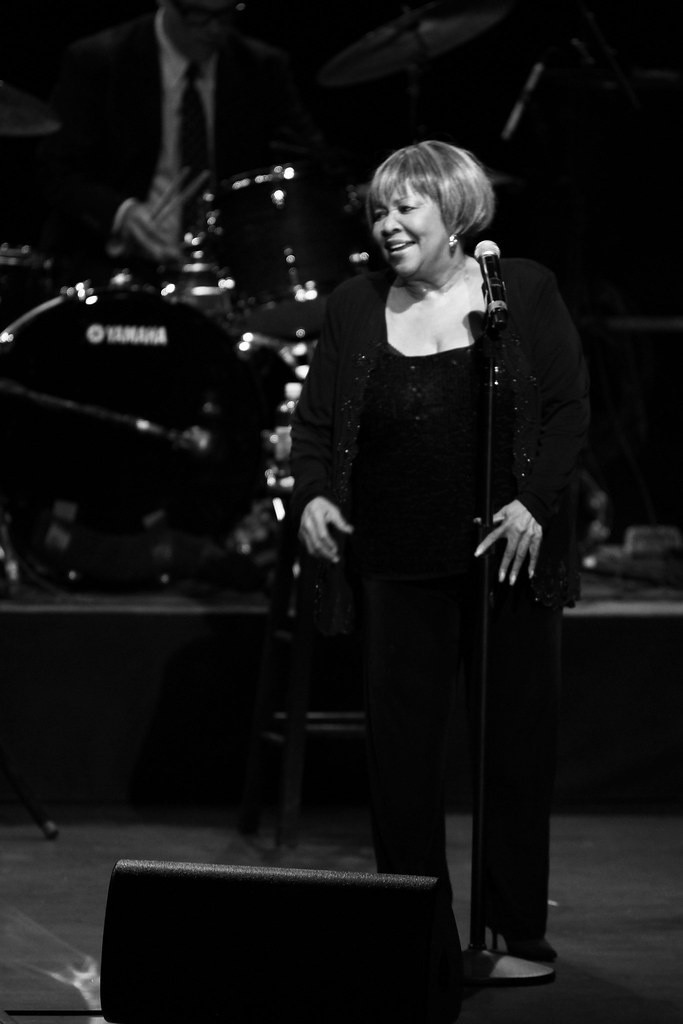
[474,239,510,334]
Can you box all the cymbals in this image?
[0,76,62,133]
[319,1,516,87]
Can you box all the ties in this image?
[176,61,212,259]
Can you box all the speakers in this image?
[99,858,466,1023]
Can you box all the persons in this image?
[37,0,327,595]
[287,140,588,961]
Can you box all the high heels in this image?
[491,931,559,963]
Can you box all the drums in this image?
[0,280,307,587]
[0,245,52,325]
[180,162,358,337]
